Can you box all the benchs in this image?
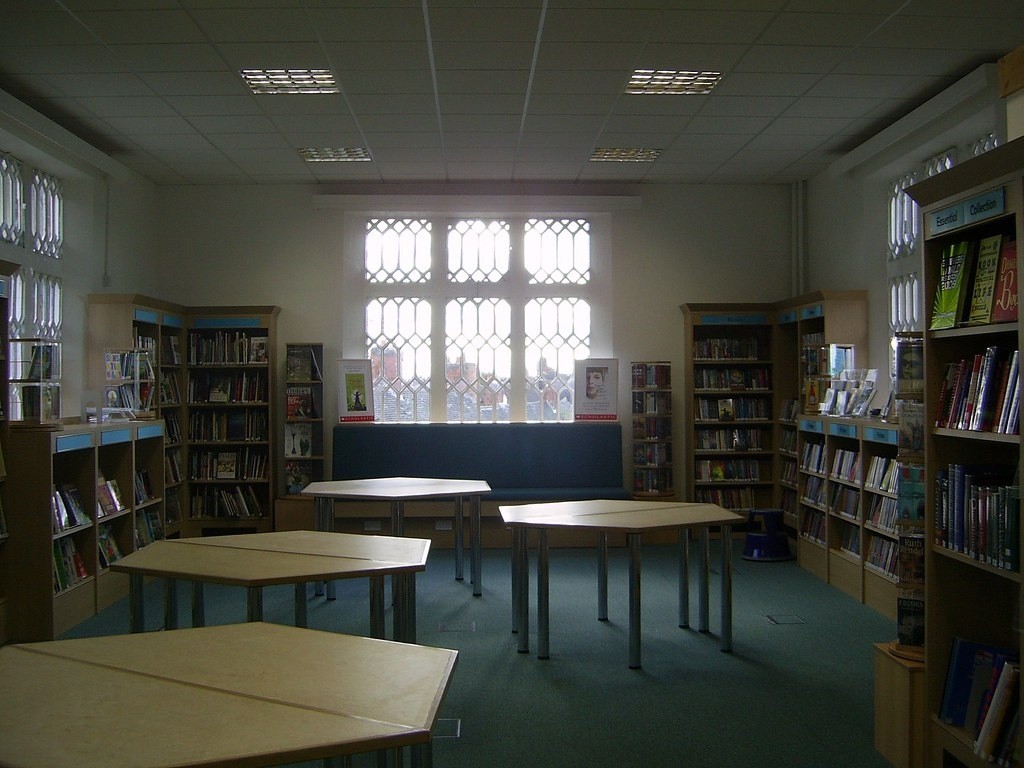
[330,423,630,551]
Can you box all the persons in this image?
[900,348,923,379]
[585,367,608,402]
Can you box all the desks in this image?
[301,476,492,602]
[0,622,459,768]
[497,498,748,670]
[109,529,430,643]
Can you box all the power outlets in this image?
[364,520,381,530]
[434,519,453,530]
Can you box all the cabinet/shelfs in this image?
[794,413,899,623]
[8,417,167,642]
[924,166,1024,768]
[81,293,282,535]
[872,325,923,768]
[677,289,870,539]
[266,342,324,532]
[630,360,683,498]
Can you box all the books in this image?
[630,360,675,496]
[49,462,164,601]
[932,344,1021,436]
[102,319,269,528]
[935,635,1023,768]
[933,461,1020,572]
[19,343,62,423]
[778,395,800,518]
[897,340,925,649]
[283,347,323,495]
[801,329,895,424]
[928,232,1020,329]
[692,331,778,531]
[799,438,900,581]
